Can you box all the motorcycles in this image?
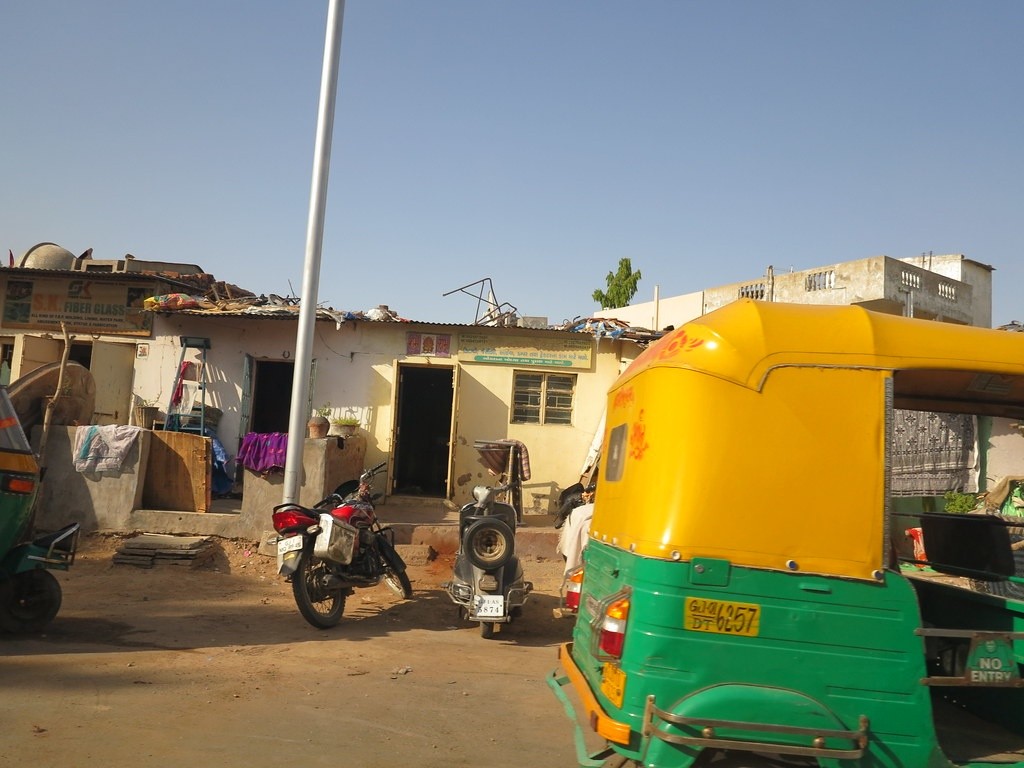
[1,387,84,631]
[270,463,419,623]
[551,303,1024,767]
[447,479,535,641]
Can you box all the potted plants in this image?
[333,417,359,436]
[132,390,162,429]
[308,402,332,440]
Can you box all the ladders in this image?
[164,334,209,433]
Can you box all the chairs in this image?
[920,512,1020,631]
